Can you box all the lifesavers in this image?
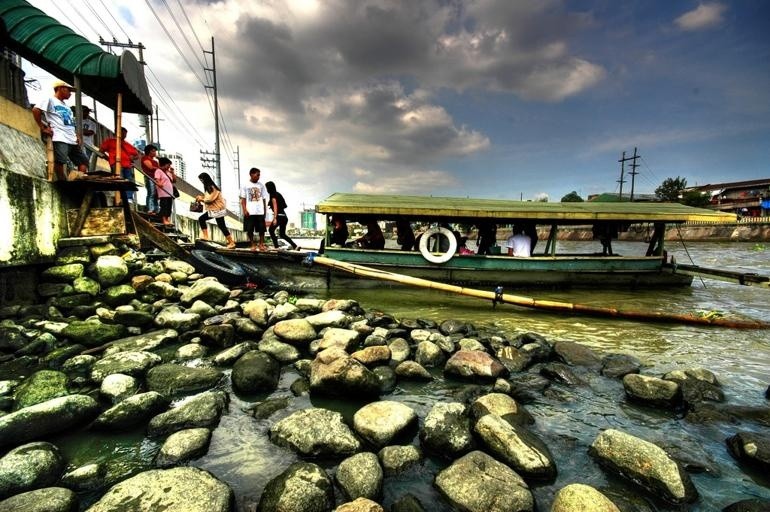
[419,228,458,263]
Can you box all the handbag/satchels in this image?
[490,246,501,254]
[190,203,203,212]
[174,185,180,197]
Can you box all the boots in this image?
[200,229,208,241]
[226,235,235,249]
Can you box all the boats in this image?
[310,192,742,294]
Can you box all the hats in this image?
[53,82,77,92]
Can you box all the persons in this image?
[141,145,176,225]
[239,168,268,252]
[264,181,301,251]
[99,127,139,203]
[32,82,96,180]
[318,219,537,257]
[195,172,236,249]
